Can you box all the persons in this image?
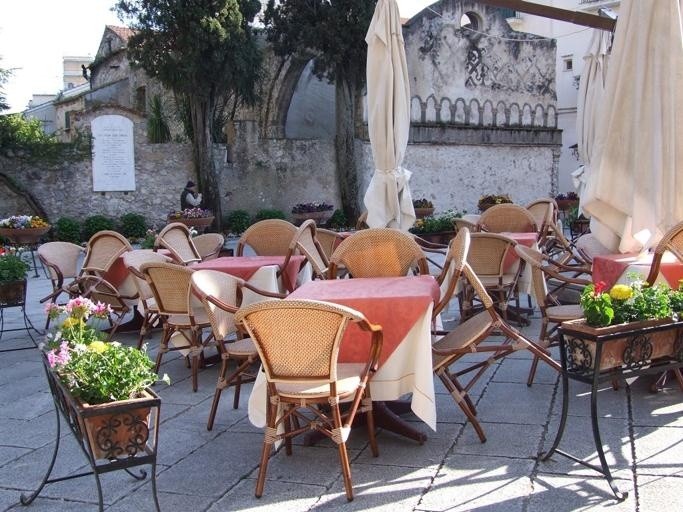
[181,181,202,211]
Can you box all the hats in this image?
[187,182,195,187]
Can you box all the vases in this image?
[0,229,50,243]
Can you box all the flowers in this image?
[0,214,50,229]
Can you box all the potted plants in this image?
[0,253,33,305]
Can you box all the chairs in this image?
[37,196,683,501]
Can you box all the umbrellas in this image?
[582,0,683,252]
[363,0,416,234]
[571,7,609,217]
